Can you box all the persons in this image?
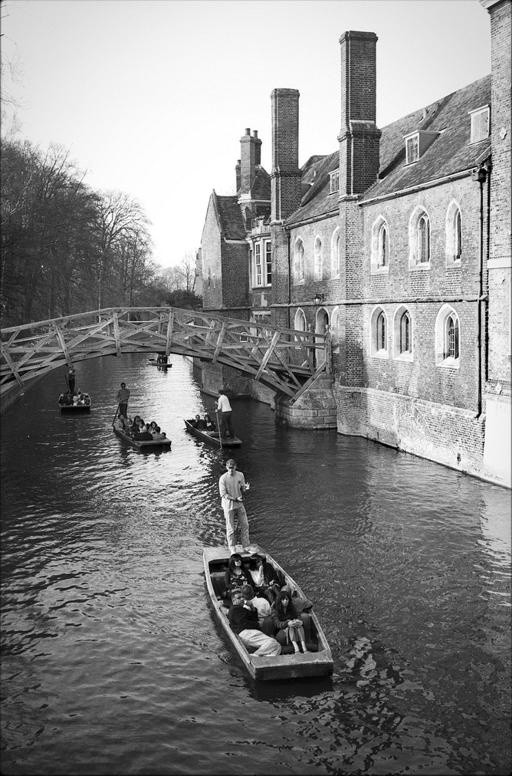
[156,351,168,363]
[204,414,216,432]
[116,382,130,419]
[58,390,90,406]
[67,364,76,394]
[219,459,251,555]
[225,553,309,657]
[192,415,207,431]
[213,389,235,440]
[115,414,166,441]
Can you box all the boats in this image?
[184,419,243,450]
[202,543,334,682]
[146,357,173,367]
[114,417,172,452]
[58,391,92,412]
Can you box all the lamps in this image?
[313,293,326,304]
